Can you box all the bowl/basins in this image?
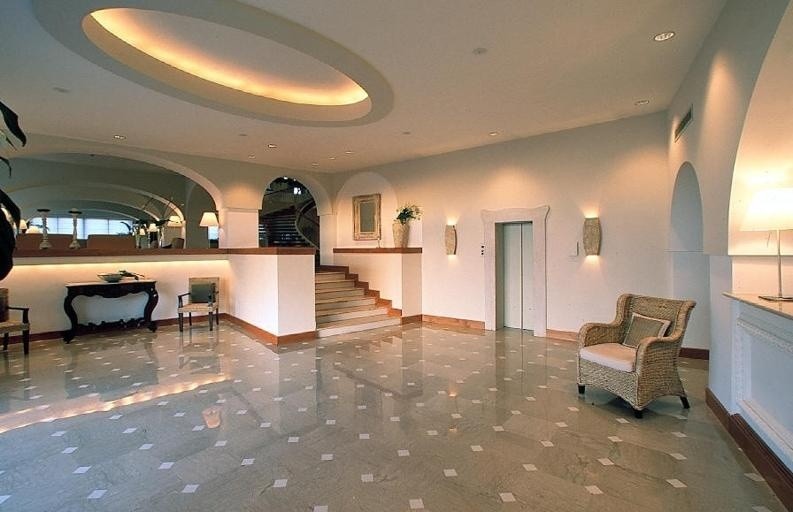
[96,274,122,283]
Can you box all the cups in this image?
[134,235,141,248]
[149,230,158,248]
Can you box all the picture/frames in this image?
[350,192,381,241]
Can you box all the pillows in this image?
[189,282,216,304]
[0,295,8,323]
[620,311,673,351]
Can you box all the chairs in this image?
[571,287,698,420]
[0,287,32,356]
[176,276,220,333]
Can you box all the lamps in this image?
[127,210,225,241]
[0,207,40,238]
[737,186,793,304]
[581,215,601,257]
[443,223,457,255]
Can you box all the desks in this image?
[60,279,160,345]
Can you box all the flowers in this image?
[389,199,424,227]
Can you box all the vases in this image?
[391,218,410,248]
